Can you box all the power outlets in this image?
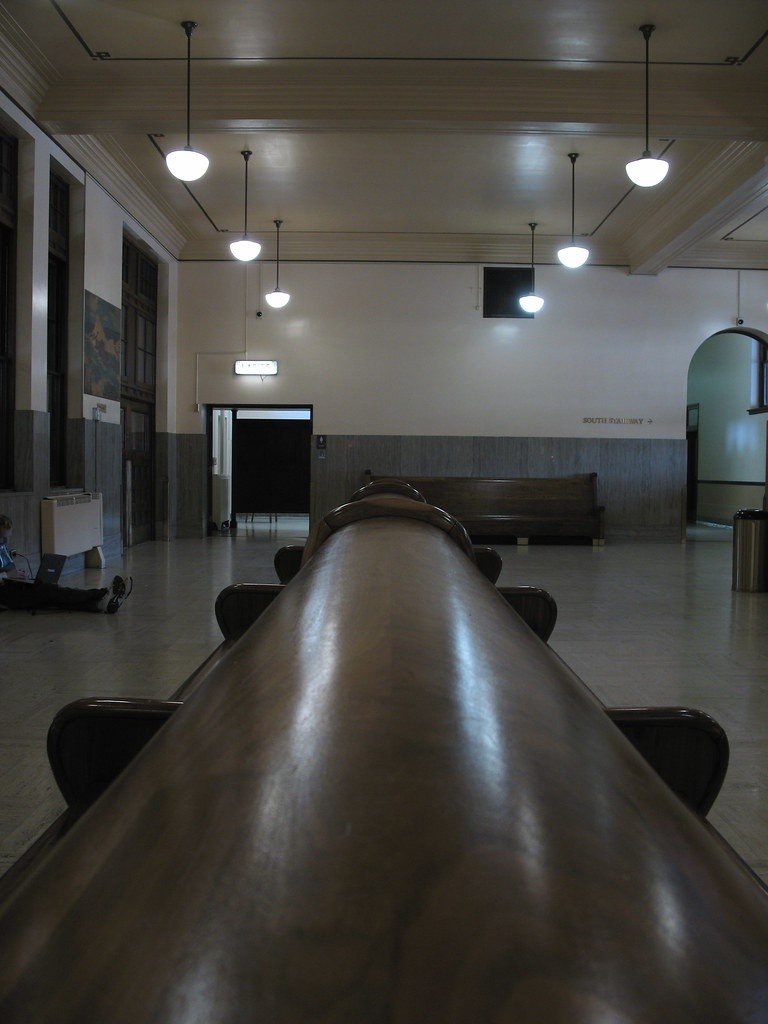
[11,550,16,560]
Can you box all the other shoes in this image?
[102,575,133,615]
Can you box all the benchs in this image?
[0,468,768,1024]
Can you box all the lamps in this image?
[625,25,669,186]
[166,21,209,181]
[230,151,261,262]
[266,220,290,308]
[519,223,544,312]
[557,153,589,268]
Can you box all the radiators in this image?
[42,492,106,568]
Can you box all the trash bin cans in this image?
[732,509,768,594]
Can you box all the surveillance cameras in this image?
[257,310,262,319]
[738,318,744,327]
